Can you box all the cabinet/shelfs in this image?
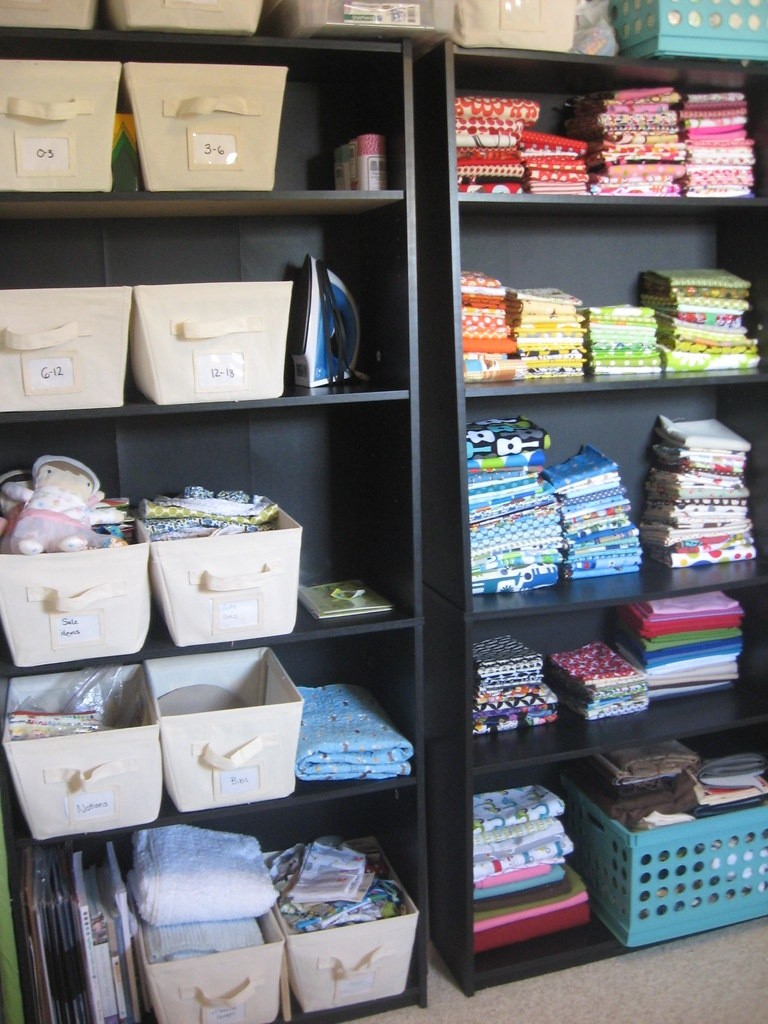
[0,26,429,1024]
[452,38,768,1008]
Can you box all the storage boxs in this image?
[608,0,768,66]
[558,770,768,948]
[104,0,263,36]
[130,279,294,407]
[123,60,290,193]
[143,648,304,812]
[0,662,164,843]
[125,879,287,1024]
[455,0,576,50]
[0,0,100,31]
[0,286,134,411]
[258,835,421,1013]
[0,59,123,193]
[137,495,304,648]
[0,507,152,668]
[264,1,455,55]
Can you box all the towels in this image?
[454,71,768,980]
[135,479,409,966]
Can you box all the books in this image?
[29,840,153,1024]
